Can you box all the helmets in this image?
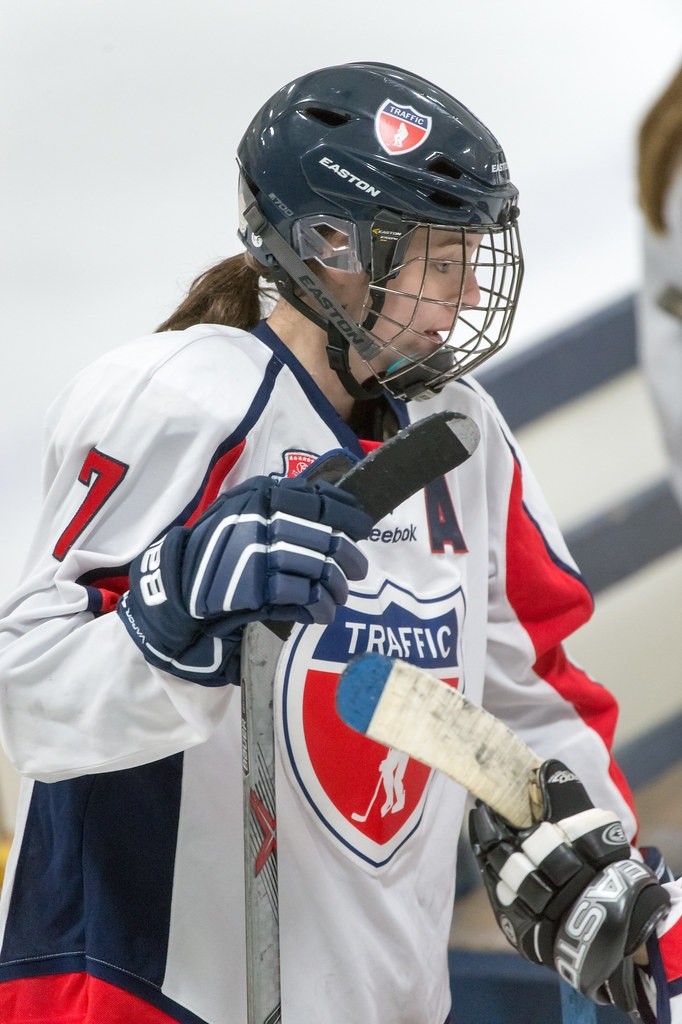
[238,62,526,400]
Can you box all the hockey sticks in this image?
[330,651,660,1024]
[237,405,482,1024]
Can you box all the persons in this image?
[1,62,682,1024]
[463,64,682,1023]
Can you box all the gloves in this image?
[118,445,374,687]
[467,760,668,1015]
